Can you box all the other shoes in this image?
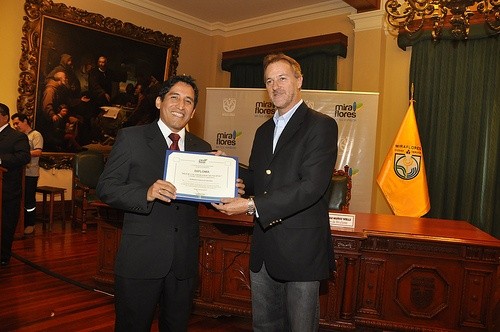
[0,255,9,266]
[24,225,35,234]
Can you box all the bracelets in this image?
[246,199,255,216]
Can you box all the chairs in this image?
[328,166,352,210]
[70,153,107,232]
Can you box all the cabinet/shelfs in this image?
[88,200,500,332]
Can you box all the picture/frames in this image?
[17,0,182,170]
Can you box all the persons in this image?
[211,55,339,332]
[11,113,44,235]
[0,103,31,265]
[96,74,245,332]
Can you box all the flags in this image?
[376,104,431,218]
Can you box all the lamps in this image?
[384,0,500,42]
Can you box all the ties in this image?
[167,132,181,152]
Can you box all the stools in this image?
[35,186,67,231]
